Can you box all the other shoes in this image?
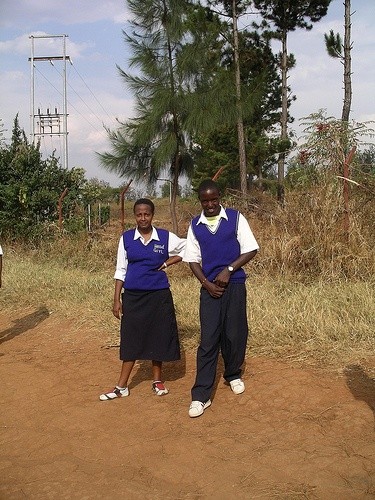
[152,380,168,395]
[100,385,129,400]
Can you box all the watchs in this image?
[227,265,235,273]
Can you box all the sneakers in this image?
[230,378,245,395]
[188,398,211,417]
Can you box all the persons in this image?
[182,180,260,418]
[0,245,3,288]
[99,198,187,401]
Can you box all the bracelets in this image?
[201,278,207,286]
[164,262,168,268]
